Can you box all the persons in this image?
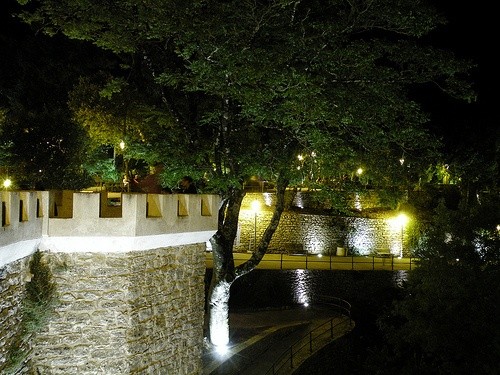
[181,174,197,194]
[122,174,131,187]
